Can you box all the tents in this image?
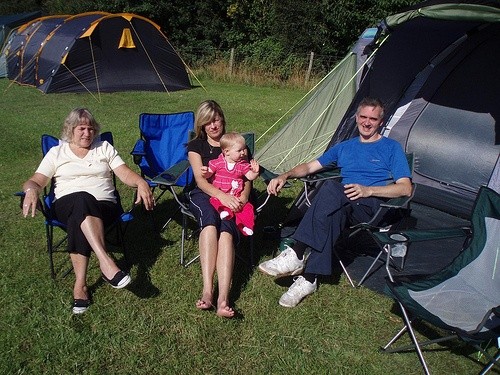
[255,4,500,354]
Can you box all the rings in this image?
[24,205,27,206]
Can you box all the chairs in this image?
[13,130,132,283]
[294,151,417,292]
[384,186,500,375]
[130,111,195,234]
[150,129,292,271]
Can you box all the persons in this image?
[183,100,260,319]
[256,97,413,306]
[0,11,192,95]
[21,108,156,314]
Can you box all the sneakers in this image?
[279,275,317,307]
[259,244,306,275]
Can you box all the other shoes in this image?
[102,266,131,289]
[73,299,90,314]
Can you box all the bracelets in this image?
[25,188,39,193]
